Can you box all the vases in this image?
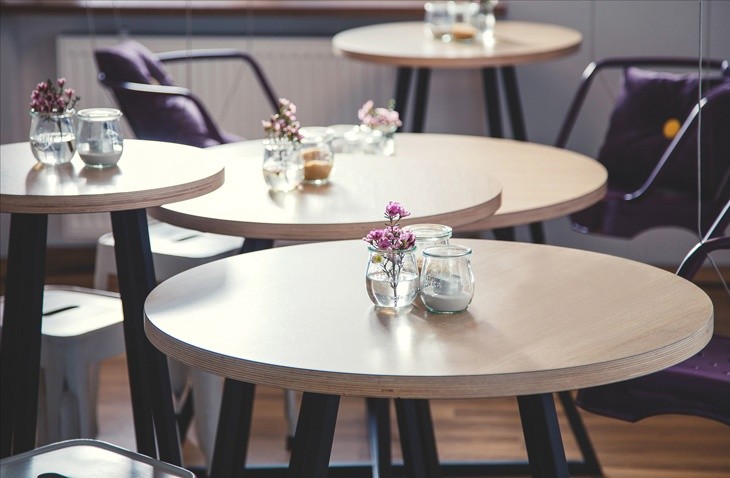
[419,244,475,315]
[356,125,402,156]
[401,223,453,309]
[299,125,335,185]
[28,109,83,165]
[449,2,478,40]
[422,2,453,41]
[260,142,306,191]
[471,0,499,39]
[362,246,421,309]
[76,107,124,167]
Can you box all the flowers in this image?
[260,96,303,184]
[30,77,74,152]
[362,200,415,307]
[357,95,402,144]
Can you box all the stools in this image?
[0,437,199,478]
[95,220,298,478]
[0,282,127,449]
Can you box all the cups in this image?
[424,1,495,41]
[261,134,334,193]
[28,108,122,168]
[366,224,475,313]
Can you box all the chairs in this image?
[93,38,284,157]
[579,234,730,430]
[557,56,730,243]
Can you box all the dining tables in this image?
[0,139,230,467]
[146,235,715,478]
[334,20,584,240]
[149,129,608,478]
